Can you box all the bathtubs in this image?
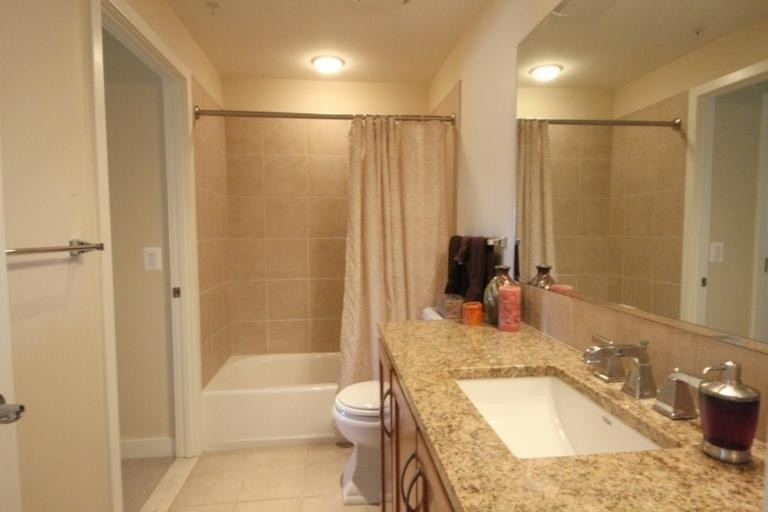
[203,351,356,446]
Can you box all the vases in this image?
[483,263,517,324]
[527,265,557,291]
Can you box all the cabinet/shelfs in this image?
[377,338,395,512]
[394,381,453,512]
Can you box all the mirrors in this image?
[514,2,768,356]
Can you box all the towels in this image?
[443,232,495,302]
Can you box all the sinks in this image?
[445,361,686,459]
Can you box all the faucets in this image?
[583,336,658,399]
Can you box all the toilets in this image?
[332,304,445,506]
